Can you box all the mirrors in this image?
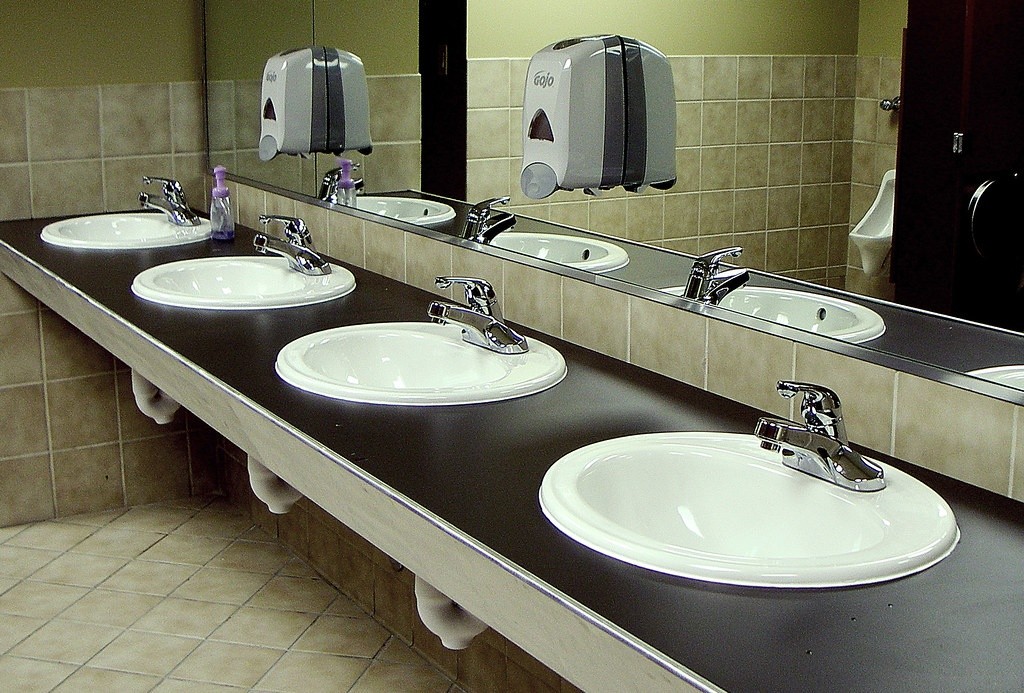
[205,0,1024,338]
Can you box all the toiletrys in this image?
[614,33,679,197]
[335,157,359,210]
[259,46,328,164]
[518,31,625,200]
[208,164,238,241]
[321,46,375,158]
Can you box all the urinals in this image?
[846,168,897,277]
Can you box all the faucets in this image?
[685,246,753,308]
[137,174,204,228]
[458,195,518,247]
[251,213,335,277]
[753,374,888,492]
[425,275,531,354]
[316,163,368,208]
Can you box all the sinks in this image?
[326,193,458,227]
[276,318,571,406]
[536,428,961,590]
[40,212,218,251]
[656,278,887,350]
[128,254,358,313]
[463,229,631,277]
